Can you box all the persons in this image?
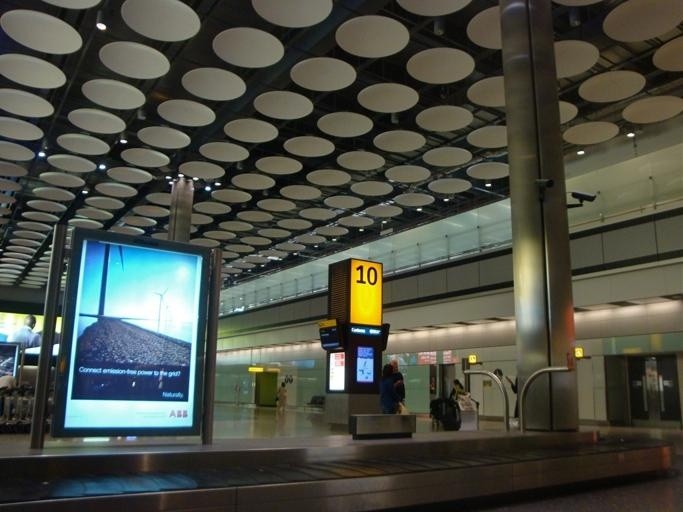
[11,314,40,350]
[379,362,402,414]
[284,374,288,384]
[449,379,464,400]
[390,358,405,401]
[289,375,293,384]
[276,381,288,414]
[504,374,519,418]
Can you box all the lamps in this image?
[432,18,445,37]
[389,113,399,124]
[94,8,107,32]
[117,107,146,146]
[36,136,49,158]
[566,5,583,30]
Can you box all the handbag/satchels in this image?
[458,393,476,411]
[429,398,461,431]
[396,402,409,415]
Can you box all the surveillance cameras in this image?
[535,179,554,188]
[571,191,597,202]
